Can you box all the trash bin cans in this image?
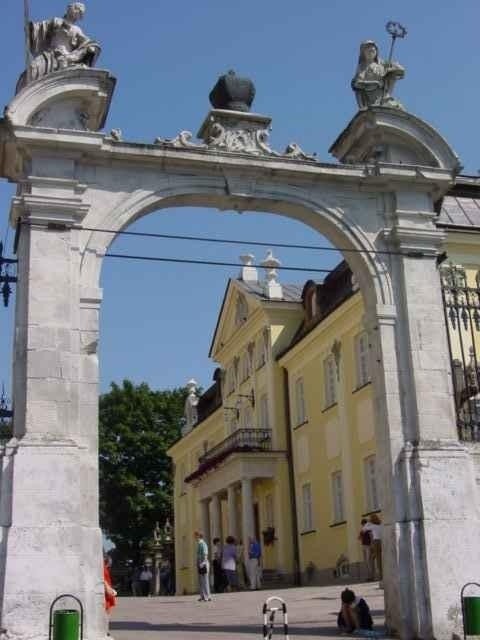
[461,582,480,640]
[49,594,83,640]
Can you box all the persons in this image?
[211,534,262,593]
[362,514,384,581]
[193,531,211,602]
[23,2,98,84]
[337,588,374,635]
[140,564,152,596]
[357,519,374,579]
[104,557,117,636]
[351,40,405,111]
